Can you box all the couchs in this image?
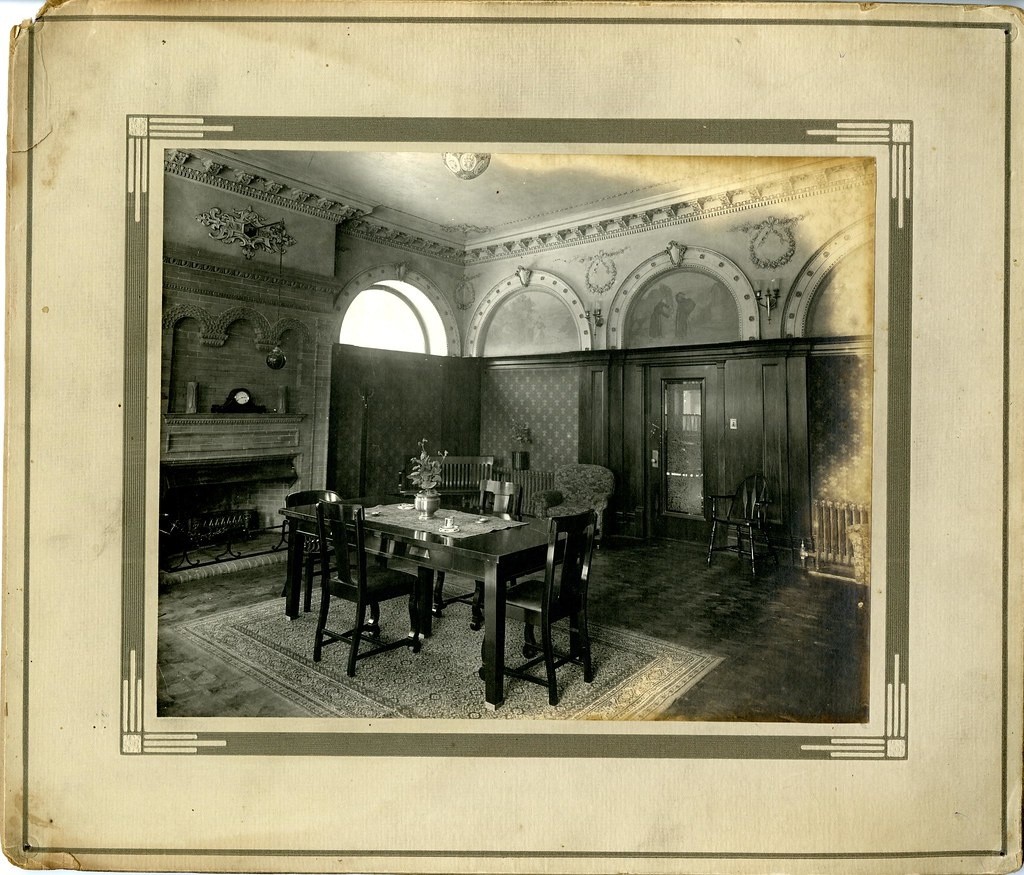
[530,464,616,551]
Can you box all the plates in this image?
[398,504,414,510]
[439,526,459,533]
[372,511,382,515]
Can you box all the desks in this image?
[278,493,600,712]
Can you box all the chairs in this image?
[313,498,423,678]
[281,489,356,613]
[705,472,780,578]
[478,508,598,706]
[432,479,523,631]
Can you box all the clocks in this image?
[211,388,268,414]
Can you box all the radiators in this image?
[813,498,871,572]
[463,465,556,518]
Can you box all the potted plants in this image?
[509,419,534,471]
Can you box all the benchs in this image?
[398,455,496,511]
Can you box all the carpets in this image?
[164,565,724,721]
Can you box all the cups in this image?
[445,517,454,529]
[444,537,454,546]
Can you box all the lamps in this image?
[753,278,781,324]
[584,299,604,340]
[242,217,287,369]
[442,152,491,180]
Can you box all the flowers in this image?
[406,438,450,496]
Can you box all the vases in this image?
[413,490,443,521]
